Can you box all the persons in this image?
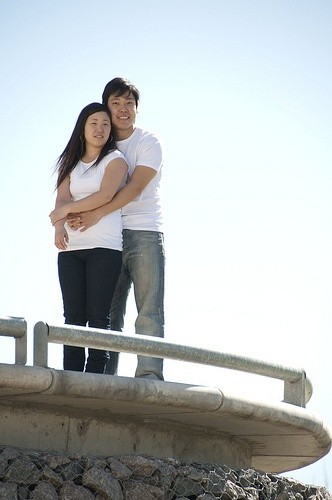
[48,102,130,374]
[102,77,165,381]
